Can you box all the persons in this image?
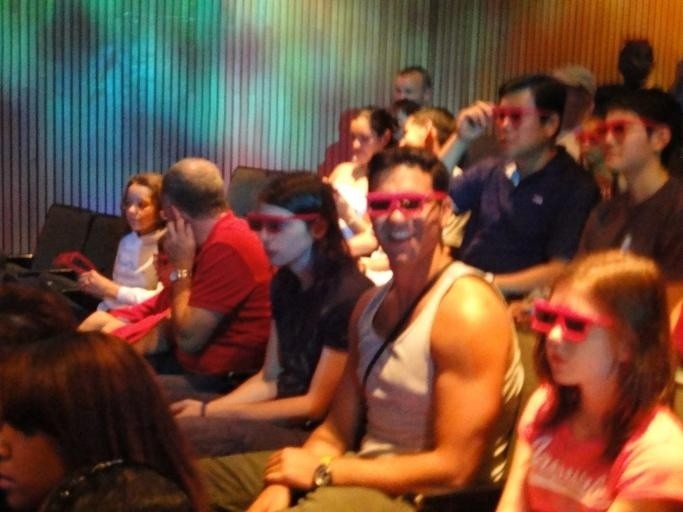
[2,39,683,512]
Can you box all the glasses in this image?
[493,106,538,129]
[246,211,319,233]
[595,120,661,144]
[366,192,446,218]
[531,301,616,345]
[53,252,94,275]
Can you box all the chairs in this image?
[0,165,290,312]
